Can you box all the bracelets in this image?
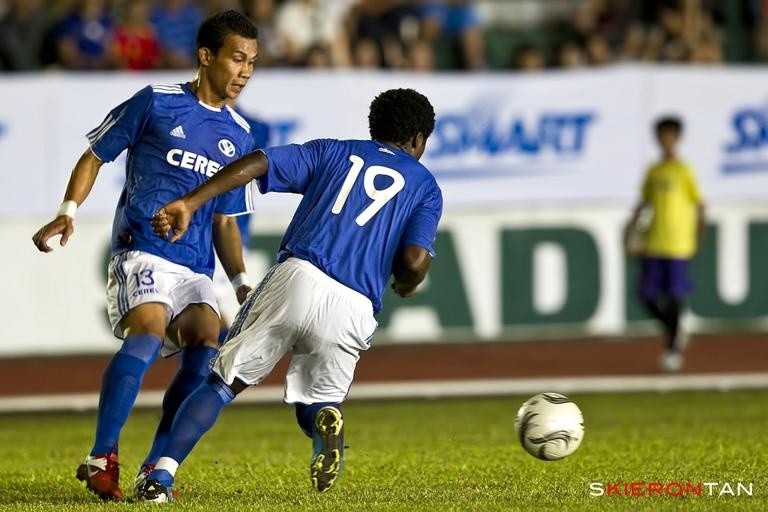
[56,199,77,219]
[230,271,252,293]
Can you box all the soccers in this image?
[516,393,583,461]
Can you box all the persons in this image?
[134,86,446,502]
[622,115,707,370]
[31,9,262,499]
[0,0,768,71]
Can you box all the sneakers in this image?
[310,405,343,493]
[76,454,124,503]
[134,464,156,481]
[125,478,169,504]
